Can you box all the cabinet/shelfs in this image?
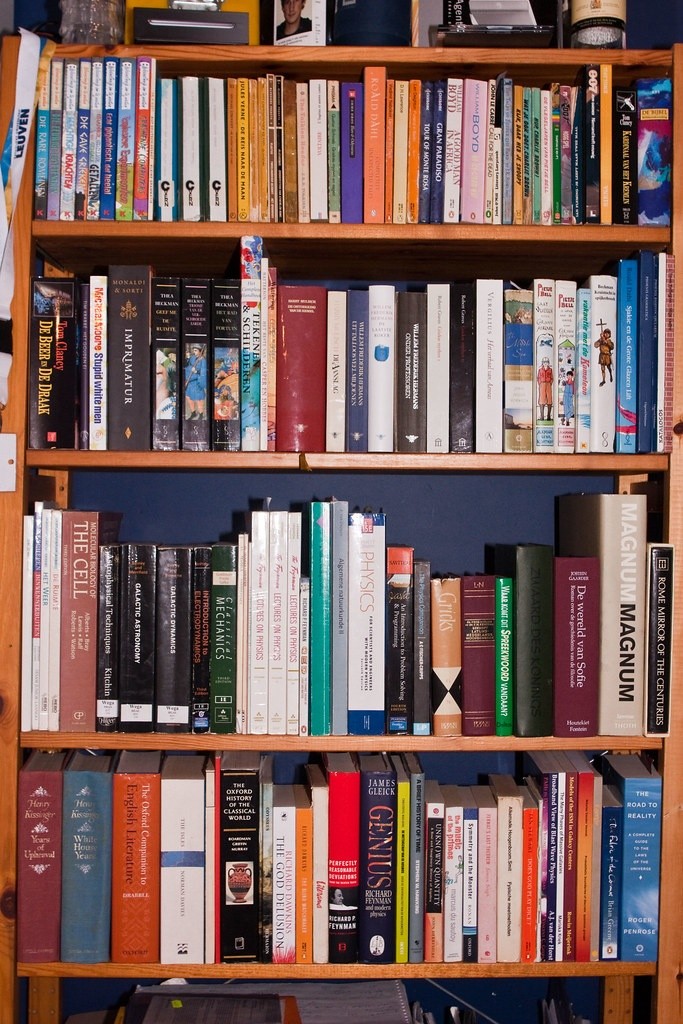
[0,33,683,1023]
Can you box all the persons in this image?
[276,0,312,38]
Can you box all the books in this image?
[38,57,671,225]
[33,235,674,455]
[116,996,302,1024]
[19,493,673,738]
[16,750,664,965]
